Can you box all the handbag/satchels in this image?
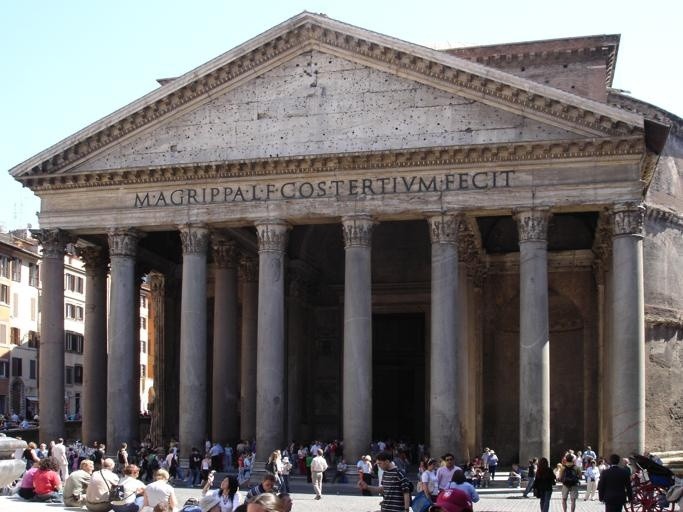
[153,502,172,512]
[416,481,435,493]
[412,491,430,512]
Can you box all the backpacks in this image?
[109,476,135,500]
[563,465,577,485]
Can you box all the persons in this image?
[1,408,82,431]
[357,440,499,512]
[508,446,663,512]
[6,437,295,512]
[298,440,348,500]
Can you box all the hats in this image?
[200,495,220,511]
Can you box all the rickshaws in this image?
[621,453,677,512]
